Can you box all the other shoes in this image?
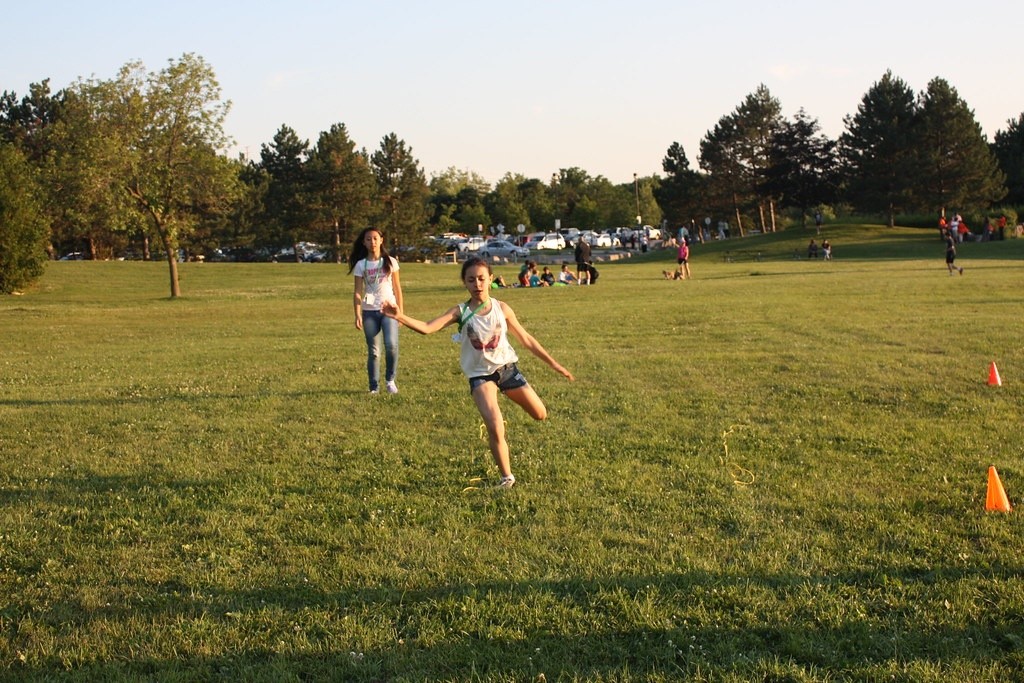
[386,381,397,393]
[959,267,963,274]
[500,476,516,490]
[371,386,380,394]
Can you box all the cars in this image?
[391,233,534,259]
[591,234,620,247]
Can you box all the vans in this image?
[524,233,566,250]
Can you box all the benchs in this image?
[720,250,767,262]
[793,246,833,261]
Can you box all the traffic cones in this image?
[986,465,1011,512]
[987,362,1001,386]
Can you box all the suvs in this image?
[560,228,580,238]
[566,232,594,246]
[611,225,661,240]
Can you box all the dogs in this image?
[672,272,683,280]
[662,271,674,279]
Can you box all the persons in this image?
[677,239,690,276]
[702,218,731,241]
[630,232,637,248]
[815,209,823,235]
[641,234,648,252]
[493,275,506,286]
[515,259,577,286]
[808,239,818,259]
[573,237,593,286]
[944,231,963,276]
[974,213,1005,243]
[821,239,831,261]
[581,260,595,284]
[939,211,969,244]
[662,232,674,248]
[346,227,403,394]
[680,225,690,246]
[379,255,574,487]
